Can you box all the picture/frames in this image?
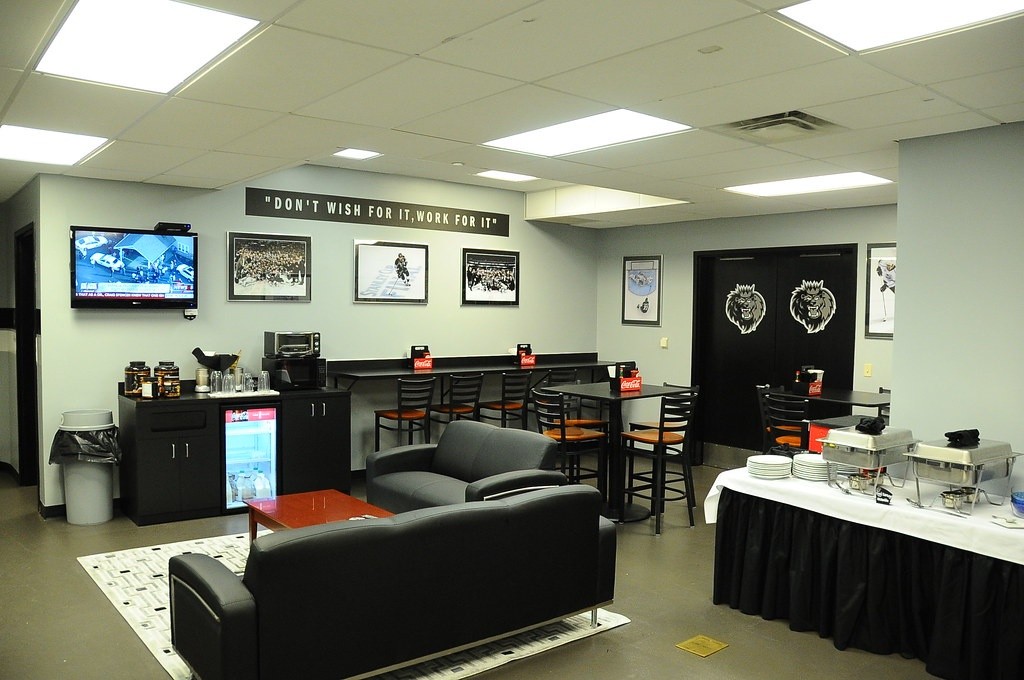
[863,242,897,340]
[226,230,312,303]
[353,238,429,305]
[462,248,520,306]
[620,255,662,327]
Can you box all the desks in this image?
[702,471,1024,680]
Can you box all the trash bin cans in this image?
[59,409,115,525]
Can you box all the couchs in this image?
[169,483,617,680]
[361,419,566,514]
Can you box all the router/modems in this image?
[154,222,192,232]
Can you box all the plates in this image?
[746,455,793,479]
[792,454,860,481]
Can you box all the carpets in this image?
[75,528,632,680]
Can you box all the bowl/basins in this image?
[1010,490,1024,519]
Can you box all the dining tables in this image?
[766,382,891,426]
[540,380,692,523]
[332,359,617,390]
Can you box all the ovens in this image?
[262,330,321,358]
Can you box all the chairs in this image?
[757,383,809,454]
[370,368,701,536]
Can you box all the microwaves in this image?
[262,357,327,391]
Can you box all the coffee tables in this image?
[242,490,396,544]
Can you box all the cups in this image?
[630,370,639,377]
[519,351,526,357]
[194,367,271,393]
[423,352,430,358]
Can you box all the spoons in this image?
[992,514,1018,523]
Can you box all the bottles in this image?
[794,371,801,384]
[619,368,624,378]
[125,361,181,399]
[227,467,271,505]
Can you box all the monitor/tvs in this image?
[70,225,198,309]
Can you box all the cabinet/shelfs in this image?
[116,380,352,527]
